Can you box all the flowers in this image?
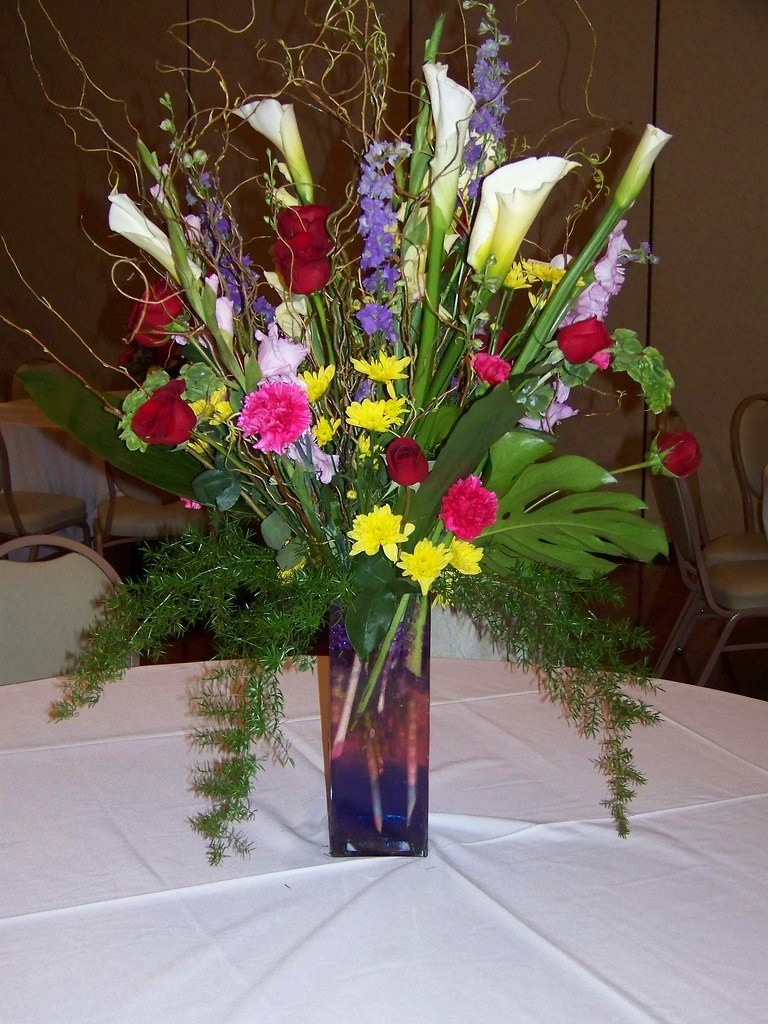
[0,0,705,873]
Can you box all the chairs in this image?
[0,432,93,560]
[92,459,222,559]
[729,394,768,533]
[651,407,768,686]
[11,358,70,404]
[0,534,142,687]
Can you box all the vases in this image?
[325,597,430,856]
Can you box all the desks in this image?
[0,655,768,1024]
[0,389,137,559]
